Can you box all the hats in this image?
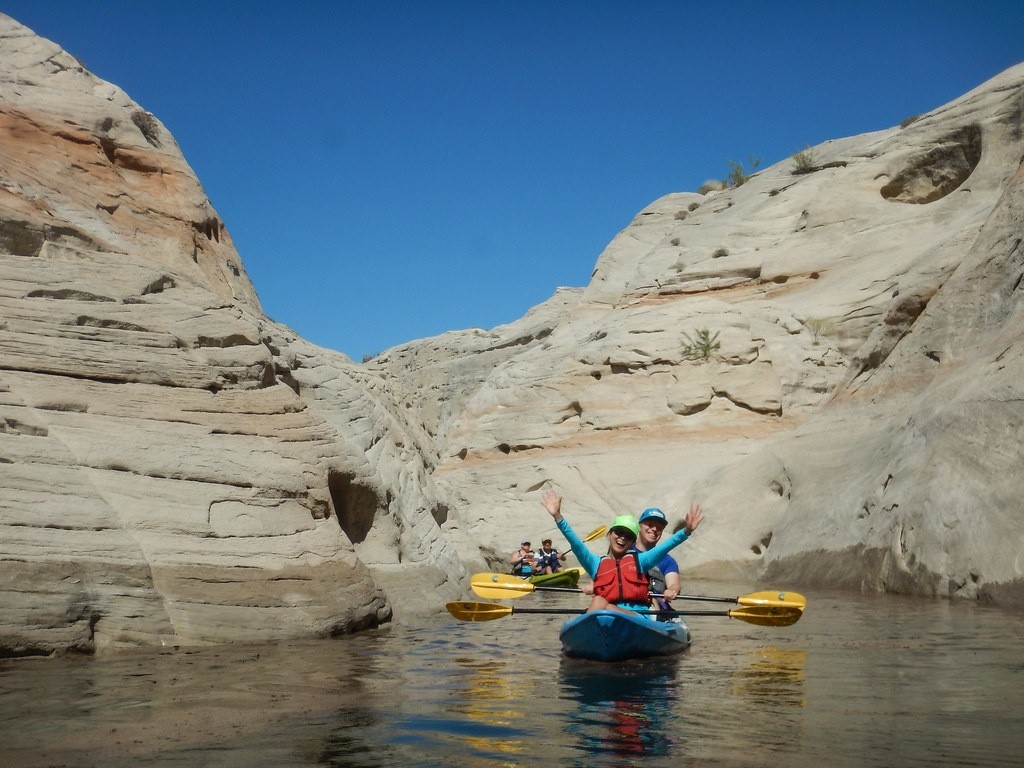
[638,508,668,527]
[542,538,552,544]
[606,515,640,540]
[521,539,530,545]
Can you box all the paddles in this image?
[469,572,806,613]
[523,524,609,580]
[444,600,805,628]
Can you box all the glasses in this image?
[611,528,634,541]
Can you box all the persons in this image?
[510,540,535,575]
[541,488,705,616]
[582,508,681,622]
[532,538,566,574]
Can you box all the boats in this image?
[558,608,693,659]
[503,568,580,589]
